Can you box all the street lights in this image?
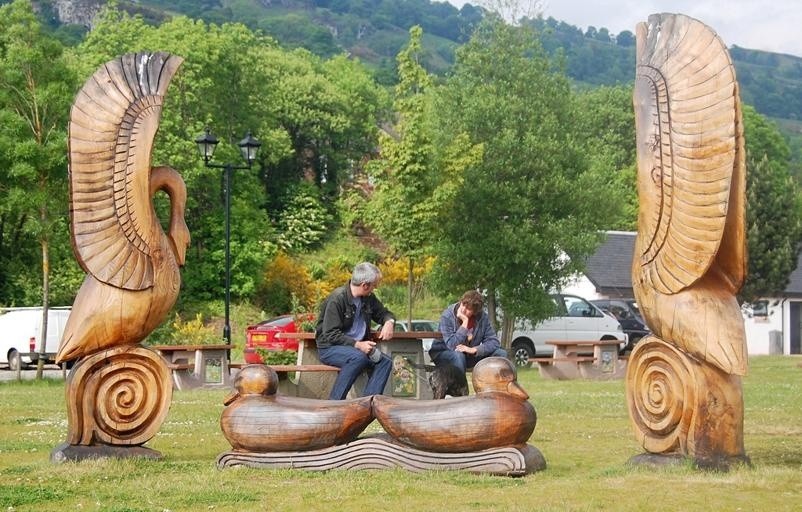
[194,125,262,375]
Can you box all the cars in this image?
[370,319,440,366]
[244,314,322,366]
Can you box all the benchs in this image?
[525,355,598,381]
[150,362,196,388]
[234,364,355,398]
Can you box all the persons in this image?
[428,290,508,372]
[315,261,396,400]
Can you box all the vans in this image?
[569,299,652,351]
[0,304,74,371]
[479,291,629,371]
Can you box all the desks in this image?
[151,344,233,390]
[544,339,625,381]
[271,331,444,400]
